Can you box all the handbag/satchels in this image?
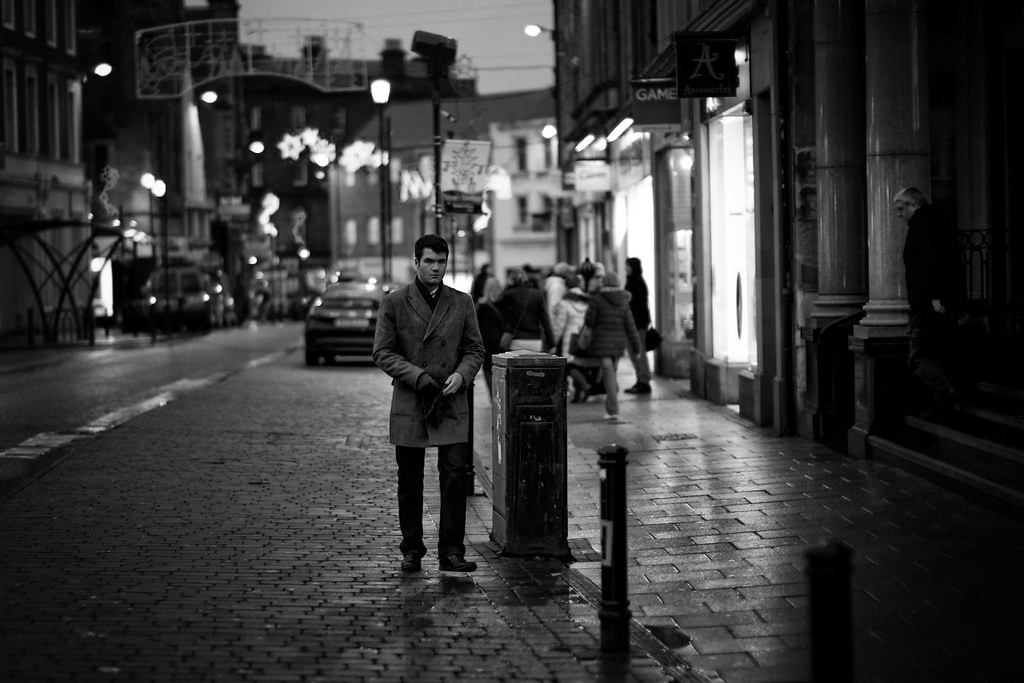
[509,339,544,352]
[500,333,514,349]
[569,324,597,359]
[644,328,662,351]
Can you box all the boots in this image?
[570,368,591,402]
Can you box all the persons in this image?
[895,188,971,414]
[373,233,485,574]
[469,258,650,421]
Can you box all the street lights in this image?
[367,76,392,284]
[141,173,166,265]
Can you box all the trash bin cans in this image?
[485,352,576,553]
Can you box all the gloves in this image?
[416,382,454,429]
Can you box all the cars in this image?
[301,282,390,367]
[140,266,237,337]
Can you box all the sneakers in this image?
[439,553,477,572]
[401,554,421,572]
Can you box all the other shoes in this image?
[571,399,579,403]
[625,381,651,394]
[605,401,618,415]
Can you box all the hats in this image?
[604,273,617,285]
